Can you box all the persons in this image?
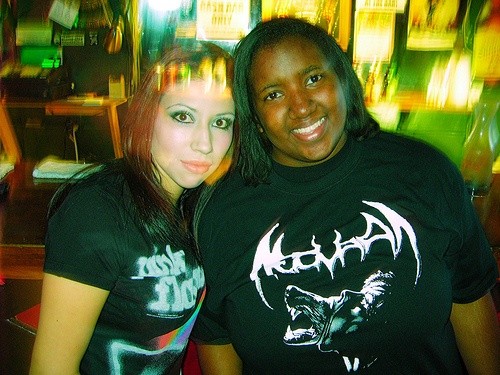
[189,16,500,374]
[27,41,235,375]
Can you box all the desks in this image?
[1,94,128,169]
